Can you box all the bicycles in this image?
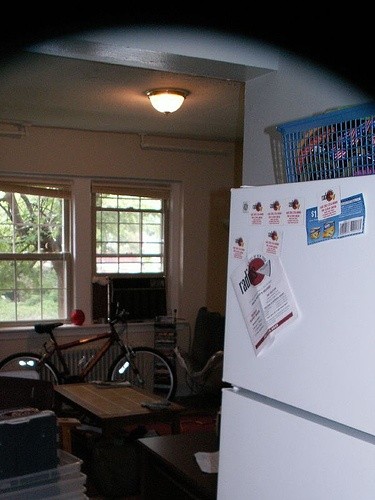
[0,301,177,416]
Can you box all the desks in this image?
[137,430,219,500]
[54,380,184,475]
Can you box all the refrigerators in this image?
[215,175,373,496]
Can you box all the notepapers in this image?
[269,200,283,225]
[316,185,341,221]
[229,245,301,358]
[231,237,247,261]
[286,197,304,225]
[250,201,264,224]
[265,228,283,259]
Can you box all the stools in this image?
[57,418,81,453]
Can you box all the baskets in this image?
[275,100,374,183]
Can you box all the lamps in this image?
[146,87,191,118]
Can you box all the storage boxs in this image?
[0,450,89,500]
[92,271,168,324]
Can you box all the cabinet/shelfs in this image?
[1,370,53,408]
[152,320,191,396]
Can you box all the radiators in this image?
[45,346,145,388]
